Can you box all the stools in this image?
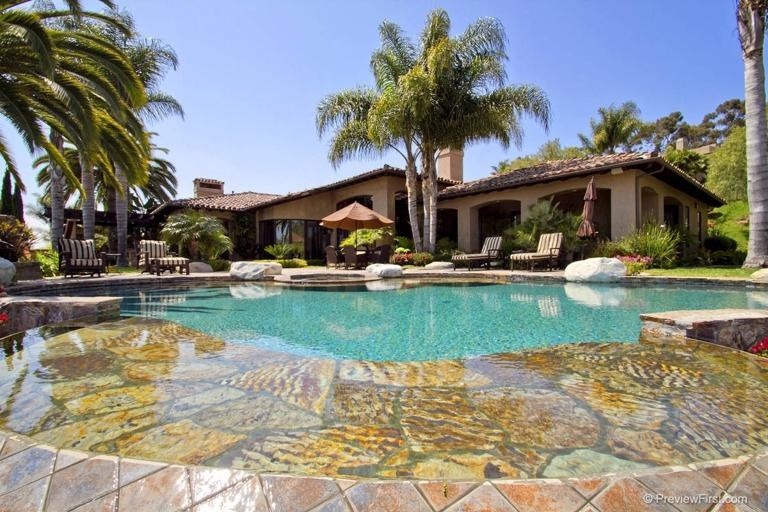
[148,257,190,275]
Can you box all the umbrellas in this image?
[574,174,597,260]
[318,200,395,251]
[319,309,395,345]
[279,230,304,247]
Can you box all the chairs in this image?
[451,236,504,271]
[510,232,566,272]
[58,237,108,277]
[325,244,393,269]
[136,239,176,274]
